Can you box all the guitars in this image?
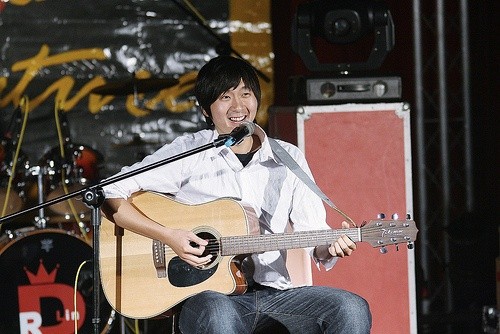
[98,190,419,319]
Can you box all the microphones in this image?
[225,120,255,148]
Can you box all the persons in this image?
[102,54,372,334]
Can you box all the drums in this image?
[0,135,31,222]
[35,143,106,215]
